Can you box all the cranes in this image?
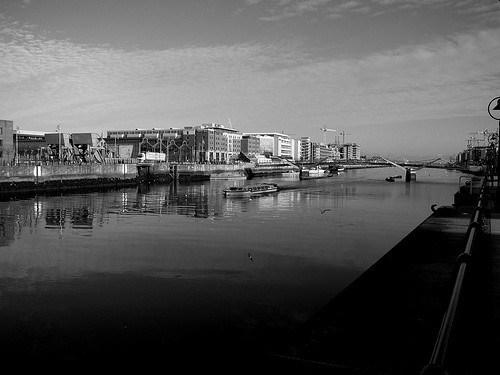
[320,126,337,142]
[339,131,352,144]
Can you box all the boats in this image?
[225,183,279,198]
[300,156,333,180]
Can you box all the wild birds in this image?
[316,207,332,215]
[247,252,254,262]
